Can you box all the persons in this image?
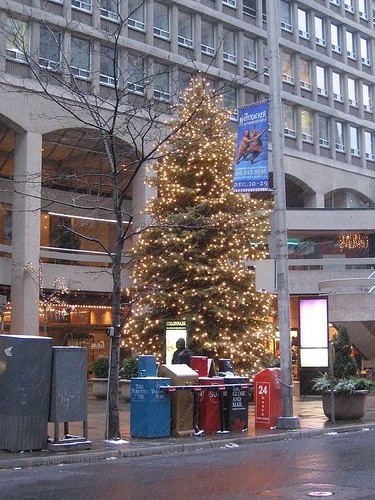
[171,338,191,368]
[236,128,267,165]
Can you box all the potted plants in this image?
[308,322,375,422]
[118,357,138,401]
[90,357,109,399]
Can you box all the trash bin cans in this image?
[190,355,224,435]
[157,362,199,438]
[219,358,253,432]
[129,353,171,439]
